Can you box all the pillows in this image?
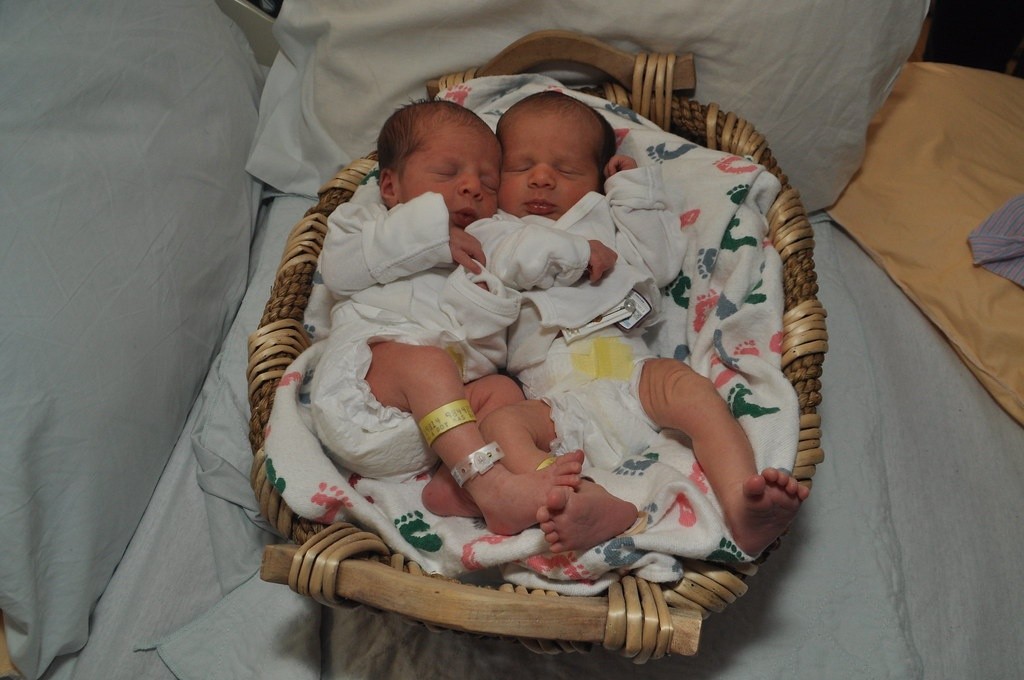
[243,0,930,215]
[1,0,268,680]
[824,64,1024,428]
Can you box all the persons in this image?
[465,90,811,558]
[310,99,584,536]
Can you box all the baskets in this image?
[247,33,830,660]
